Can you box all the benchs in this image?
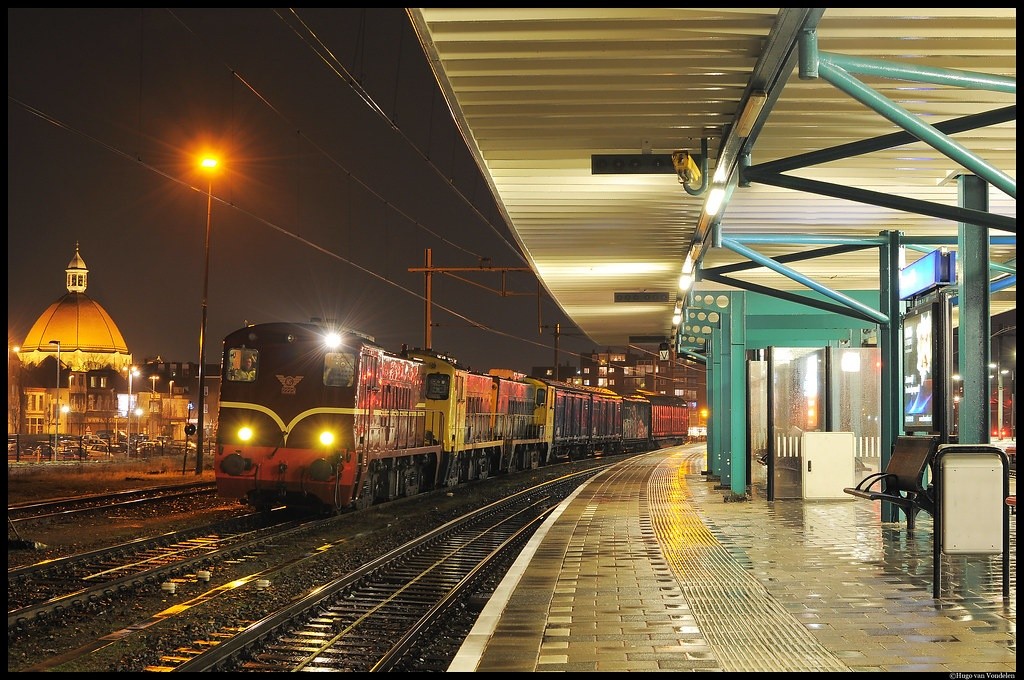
[843,434,937,530]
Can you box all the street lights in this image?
[135,409,143,436]
[120,363,141,458]
[147,373,160,442]
[49,341,60,462]
[169,379,175,436]
[194,147,218,472]
[62,406,68,432]
[121,410,127,436]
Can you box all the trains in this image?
[215,317,690,514]
